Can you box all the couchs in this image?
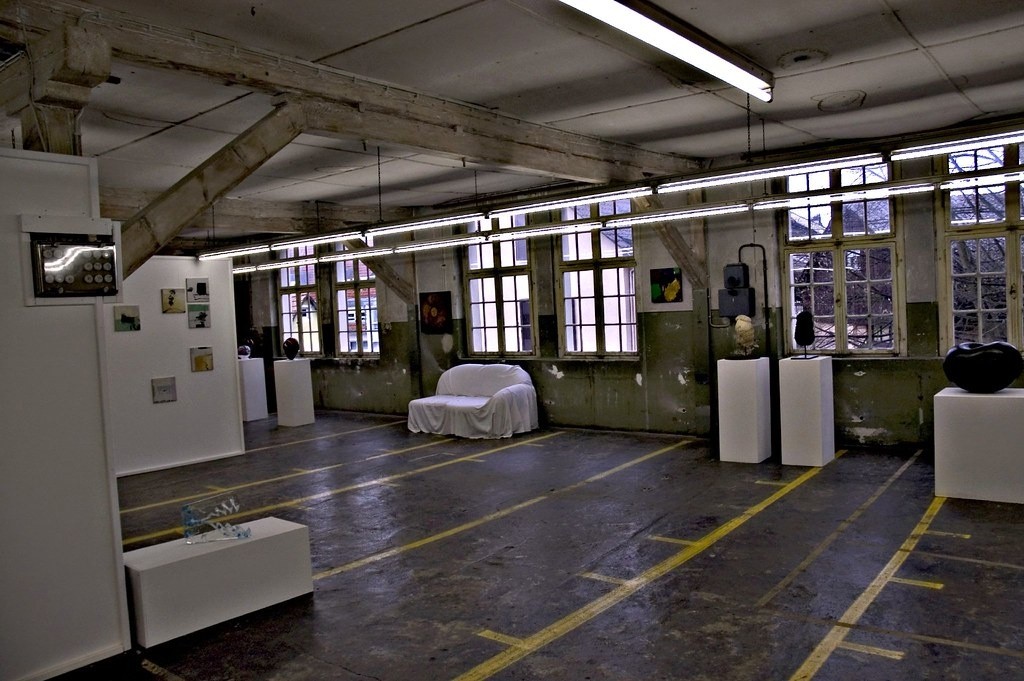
[408,364,539,442]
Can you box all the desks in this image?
[119,515,315,660]
[934,387,1024,505]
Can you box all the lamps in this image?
[557,0,775,105]
